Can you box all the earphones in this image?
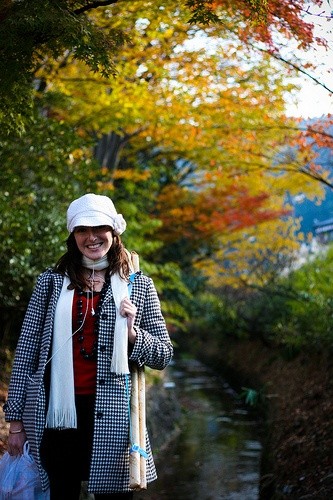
[91,308,95,316]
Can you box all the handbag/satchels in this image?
[0,440,52,500]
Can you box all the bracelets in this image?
[9,429,23,434]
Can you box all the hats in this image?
[66,192,128,237]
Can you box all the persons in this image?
[3,194,173,500]
